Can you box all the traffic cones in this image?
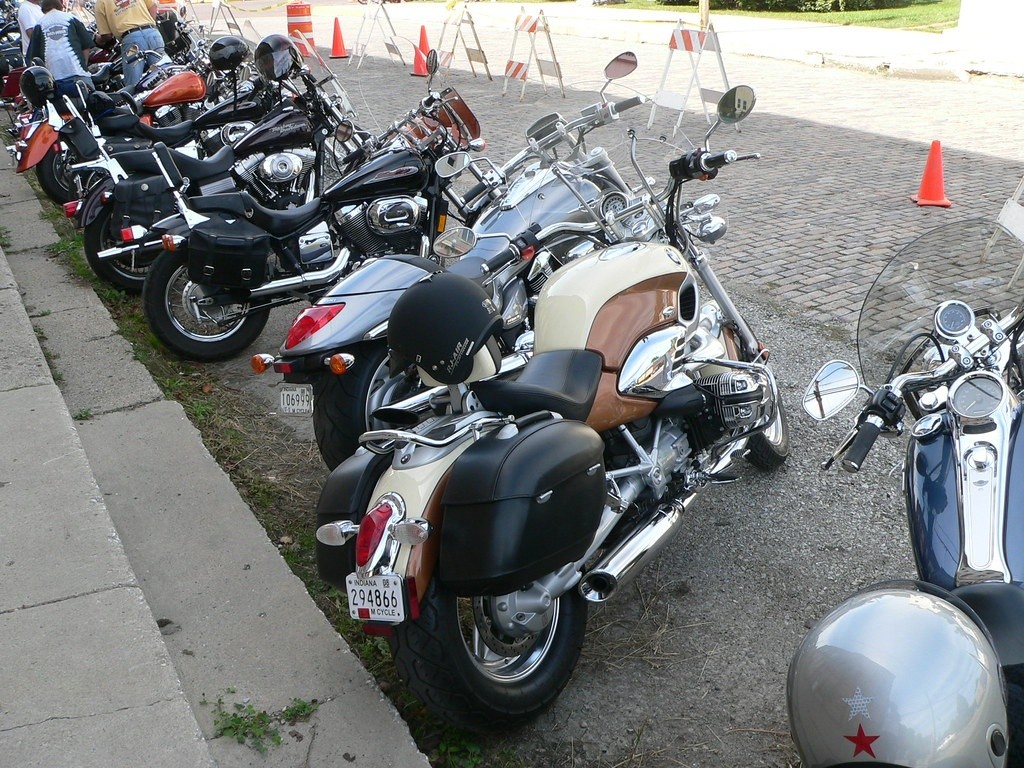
[909,140,951,207]
[327,16,349,59]
[409,24,432,76]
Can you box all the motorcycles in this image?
[1,1,791,720]
[786,214,1024,768]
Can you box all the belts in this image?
[121,25,156,39]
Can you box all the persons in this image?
[93,0,164,88]
[25,0,95,97]
[18,0,45,64]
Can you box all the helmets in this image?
[385,272,503,384]
[786,587,1010,768]
[20,66,53,105]
[254,34,305,80]
[207,35,249,69]
